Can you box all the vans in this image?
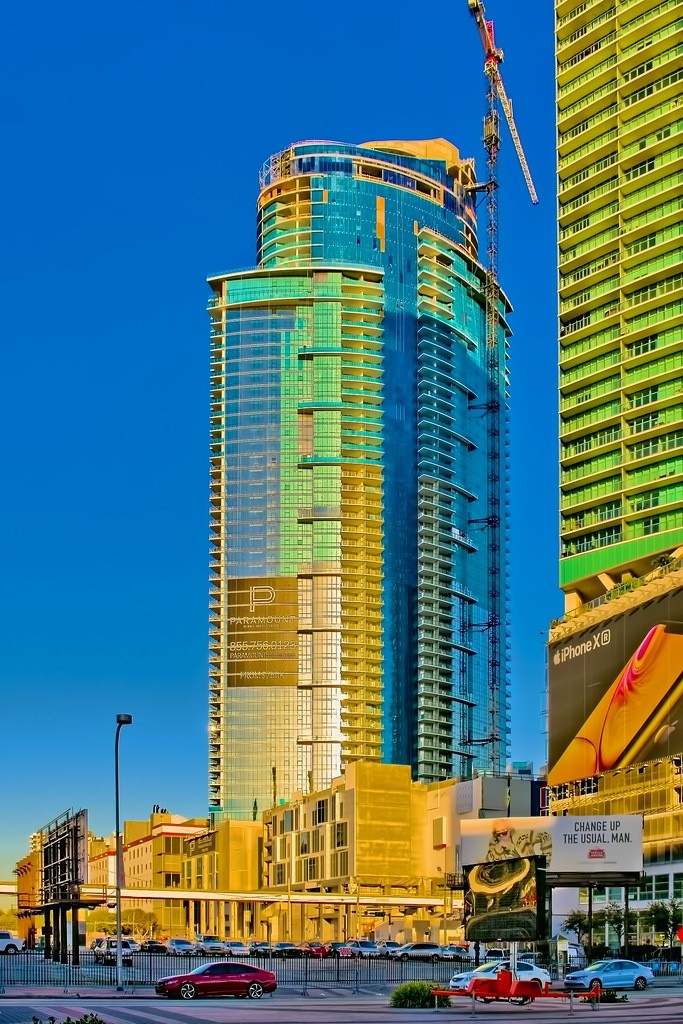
[0,930,13,939]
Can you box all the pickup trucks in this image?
[0,939,27,955]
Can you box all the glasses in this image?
[496,830,508,837]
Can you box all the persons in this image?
[485,819,552,869]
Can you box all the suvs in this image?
[375,939,401,960]
[485,948,510,962]
[518,952,544,964]
[391,943,444,963]
[195,935,225,957]
[344,940,381,959]
[93,939,133,967]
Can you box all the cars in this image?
[323,941,347,959]
[563,959,654,991]
[140,940,166,953]
[300,941,326,958]
[274,942,303,959]
[439,945,471,962]
[449,960,552,991]
[224,941,250,958]
[154,962,278,1000]
[164,938,197,957]
[123,939,141,954]
[248,941,276,959]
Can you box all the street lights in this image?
[114,713,133,991]
